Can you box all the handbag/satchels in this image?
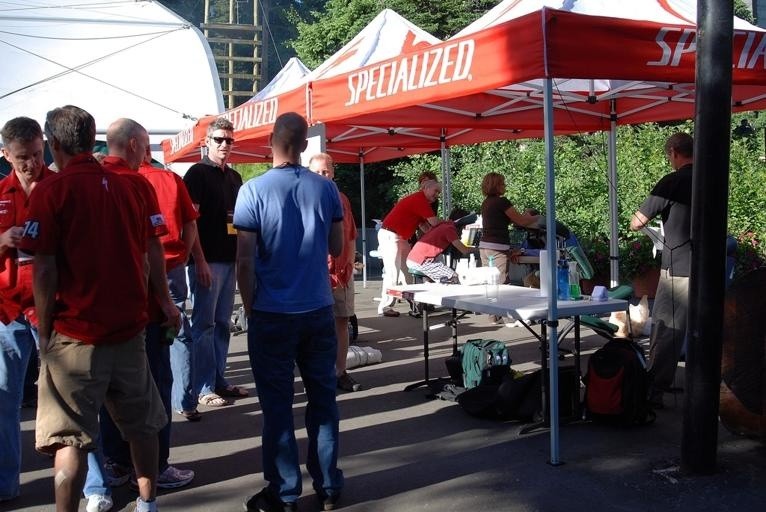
[460,336,511,387]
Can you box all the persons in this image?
[234,109,344,512]
[307,154,362,394]
[47,120,199,417]
[98,117,195,491]
[45,106,157,512]
[376,172,541,318]
[630,133,692,395]
[181,119,247,407]
[0,116,60,512]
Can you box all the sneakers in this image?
[338,371,361,391]
[104,460,133,486]
[242,487,297,511]
[383,306,400,316]
[86,493,115,512]
[130,464,194,492]
[179,409,202,420]
[317,493,339,510]
[507,318,531,328]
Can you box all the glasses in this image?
[208,134,234,145]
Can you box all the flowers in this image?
[619,232,661,282]
[581,233,609,272]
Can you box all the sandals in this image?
[198,393,226,406]
[222,385,248,398]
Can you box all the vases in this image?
[581,270,603,296]
[633,268,659,298]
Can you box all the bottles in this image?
[556,258,570,301]
[468,253,477,269]
[567,260,580,301]
[160,322,176,346]
[494,355,508,365]
[485,256,501,304]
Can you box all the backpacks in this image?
[585,336,659,426]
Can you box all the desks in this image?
[385,284,628,435]
